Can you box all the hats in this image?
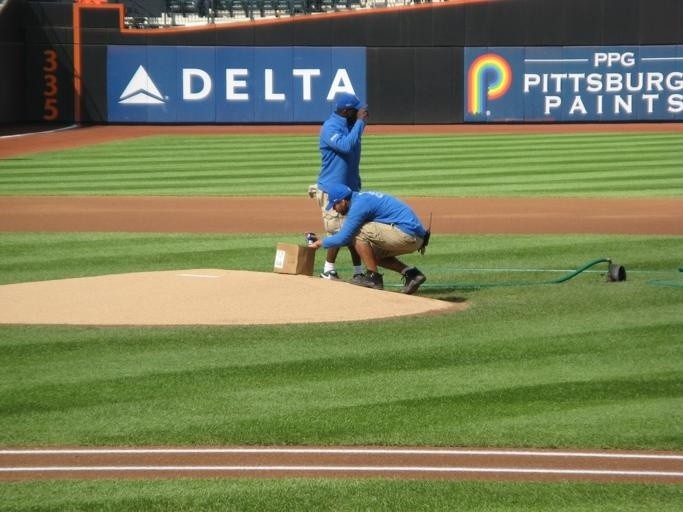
[325,183,352,210]
[336,93,368,110]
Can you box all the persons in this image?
[308,93,371,281]
[308,182,427,294]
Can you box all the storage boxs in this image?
[273,242,316,276]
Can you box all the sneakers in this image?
[350,269,383,290]
[400,268,426,294]
[320,270,343,282]
